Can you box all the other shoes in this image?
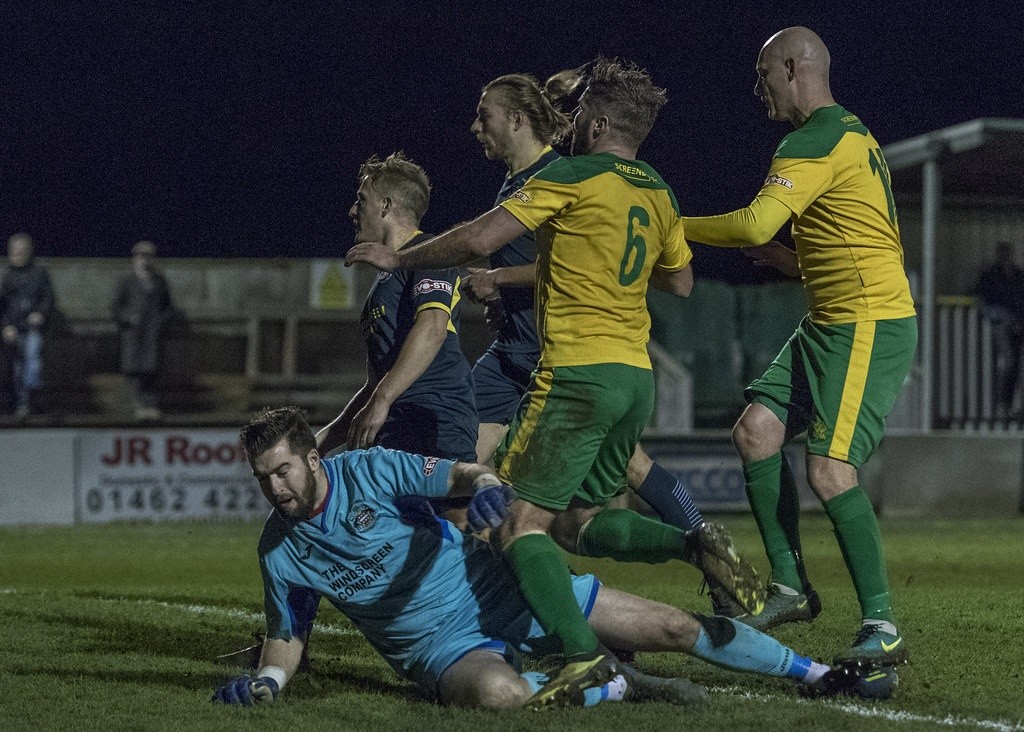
[16,405,30,421]
[134,407,161,424]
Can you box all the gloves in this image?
[208,665,286,708]
[465,473,517,533]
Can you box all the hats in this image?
[131,241,156,255]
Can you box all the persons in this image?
[1,230,66,428]
[460,70,743,617]
[247,152,482,671]
[974,243,1023,432]
[345,56,766,728]
[107,240,175,420]
[682,25,920,670]
[210,406,901,711]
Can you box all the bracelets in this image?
[257,665,290,692]
[472,473,503,491]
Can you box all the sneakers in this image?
[214,633,312,673]
[682,521,765,617]
[523,640,620,713]
[798,656,899,701]
[833,624,908,676]
[732,583,821,631]
[698,572,746,619]
[619,664,710,705]
